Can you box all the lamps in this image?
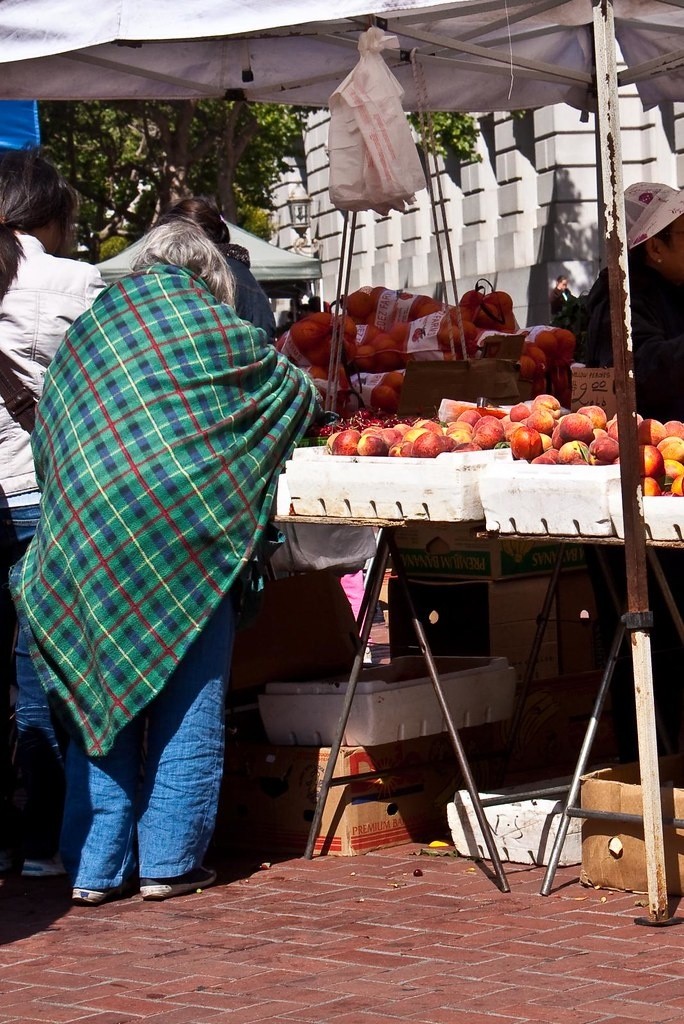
[285,181,324,261]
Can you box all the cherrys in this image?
[306,408,439,438]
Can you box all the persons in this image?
[1,140,386,907]
[552,182,684,766]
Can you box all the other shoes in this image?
[140,866,217,899]
[71,882,129,904]
[0,848,23,872]
[22,860,66,876]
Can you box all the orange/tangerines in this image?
[278,286,575,410]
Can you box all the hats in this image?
[623,181,684,250]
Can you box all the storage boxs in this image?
[210,439,684,899]
[396,335,526,419]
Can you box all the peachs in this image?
[327,394,684,496]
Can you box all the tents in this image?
[1,1,684,929]
[95,216,323,323]
